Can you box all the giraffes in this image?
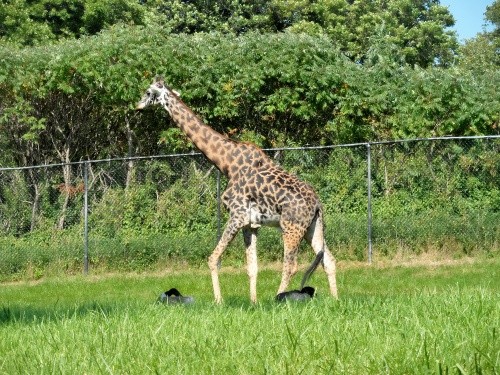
[137,74,338,303]
